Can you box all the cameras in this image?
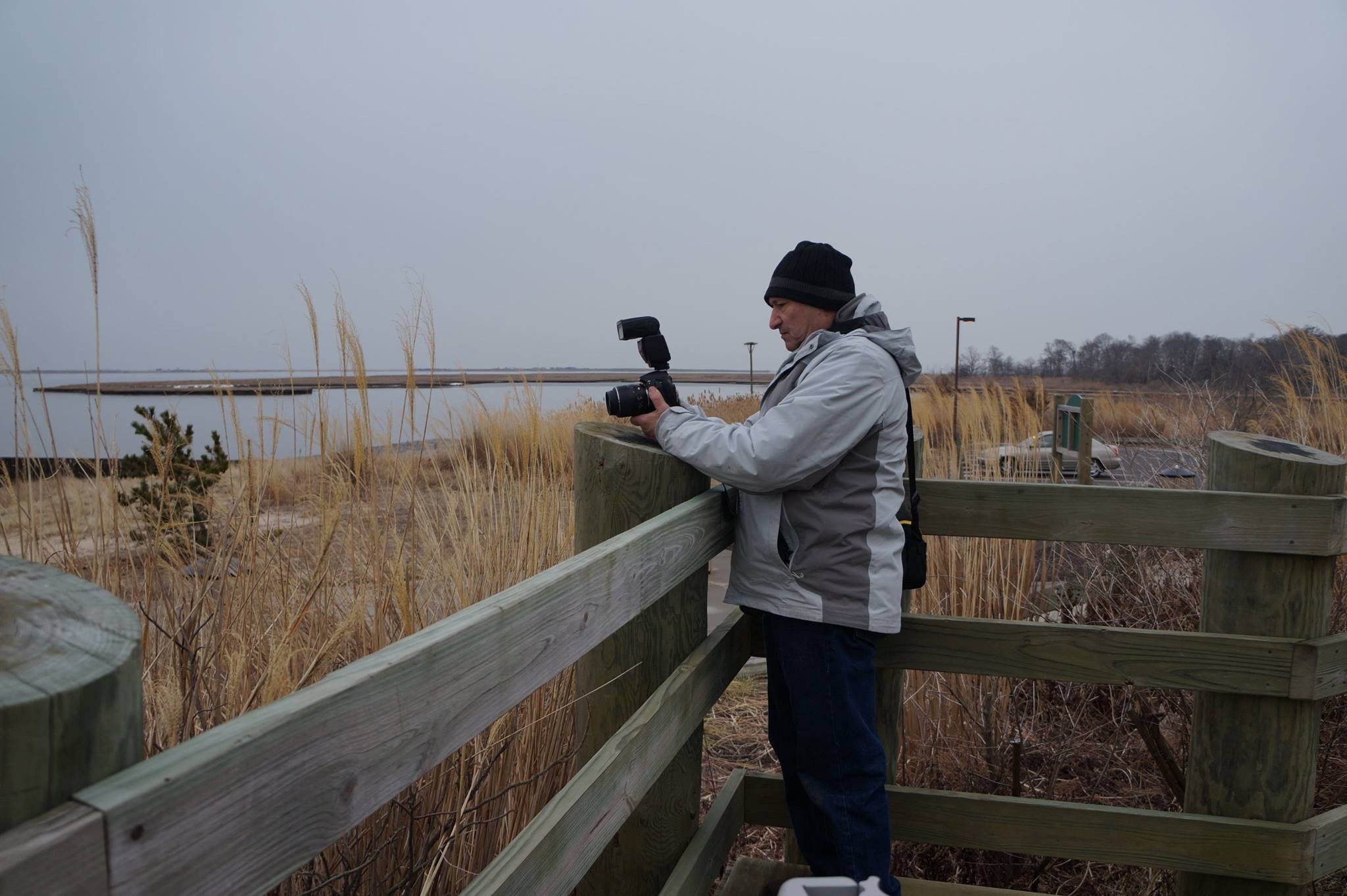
[606,315,681,418]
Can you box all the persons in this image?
[632,240,923,896]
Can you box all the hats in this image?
[764,241,856,311]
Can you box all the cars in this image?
[975,430,1120,478]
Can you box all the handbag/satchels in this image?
[894,510,927,591]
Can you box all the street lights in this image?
[745,341,758,401]
[952,316,975,440]
